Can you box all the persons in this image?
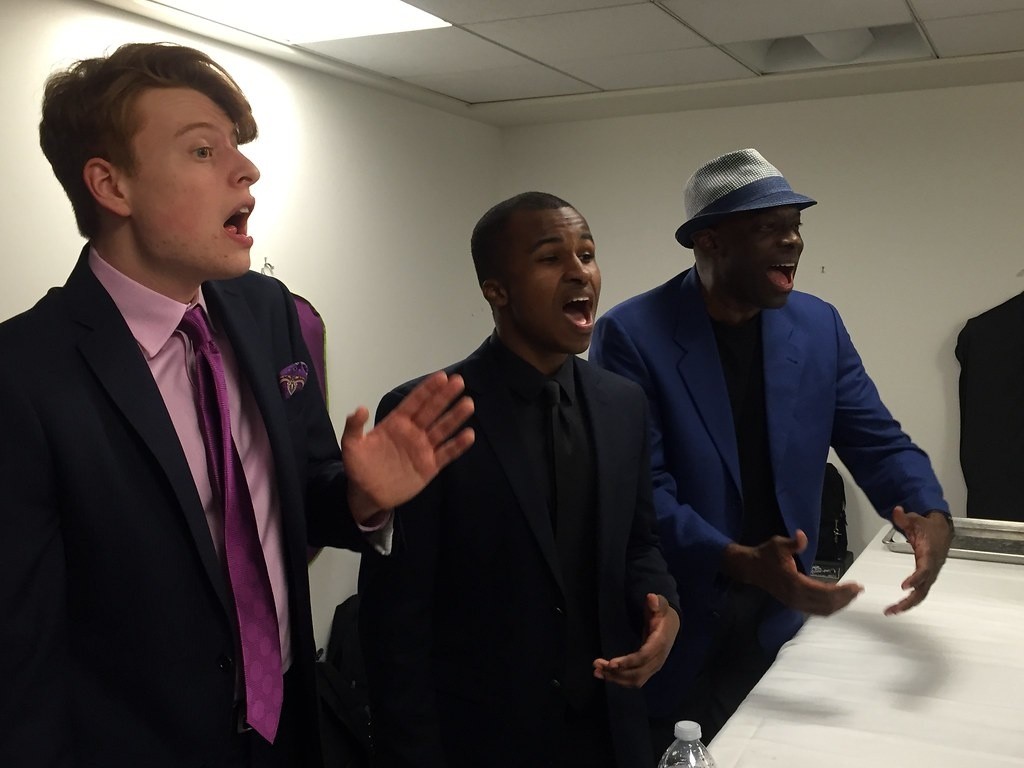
[0,41,483,768]
[365,190,687,768]
[588,147,957,764]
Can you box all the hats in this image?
[675,148,820,250]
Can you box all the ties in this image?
[178,304,284,745]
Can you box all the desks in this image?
[701,517,1024,768]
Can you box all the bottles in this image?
[658,720,717,768]
